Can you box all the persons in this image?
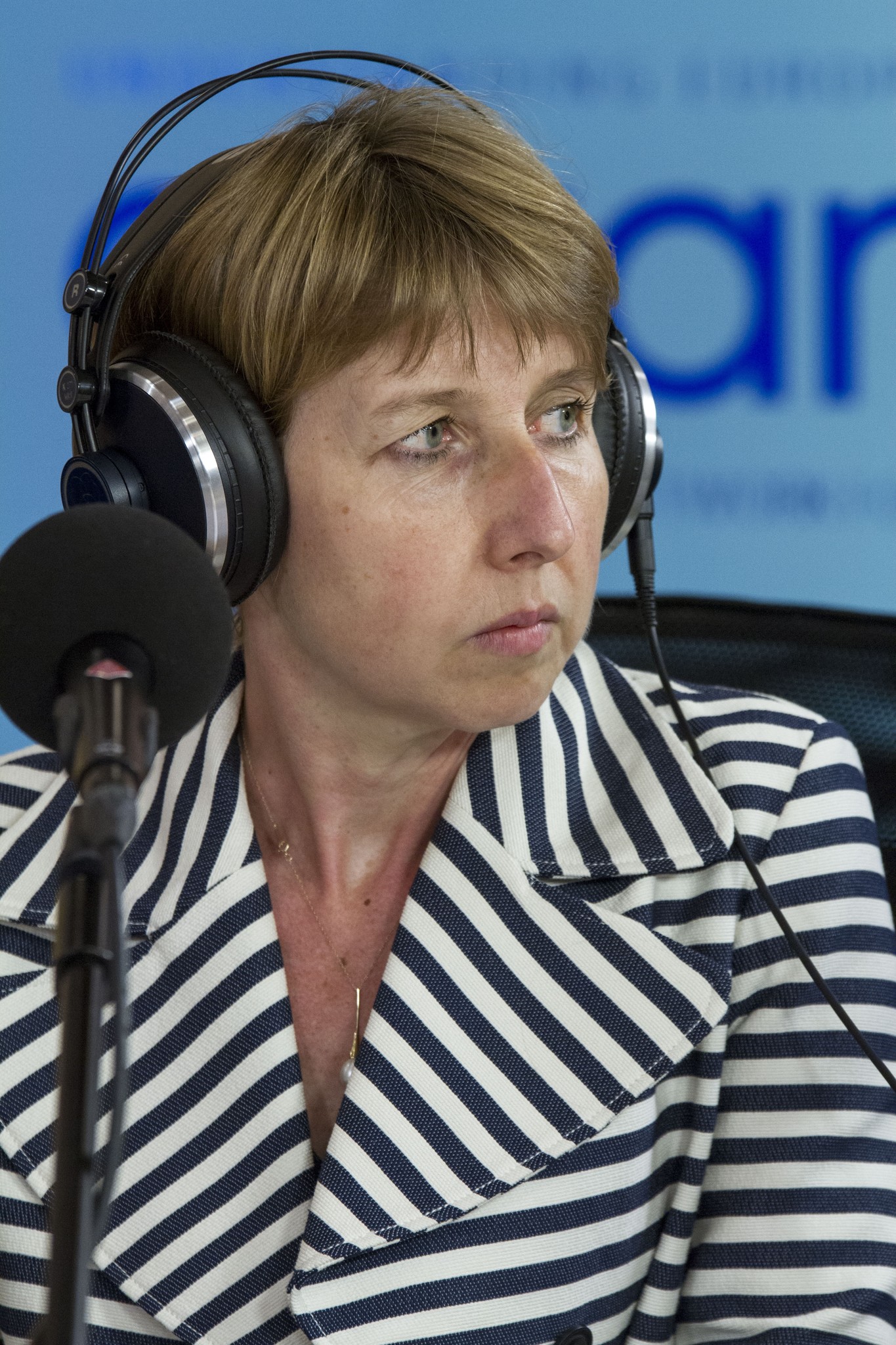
[0,85,896,1345]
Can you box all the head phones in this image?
[55,51,666,603]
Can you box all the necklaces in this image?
[237,736,394,1075]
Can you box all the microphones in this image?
[0,504,232,851]
